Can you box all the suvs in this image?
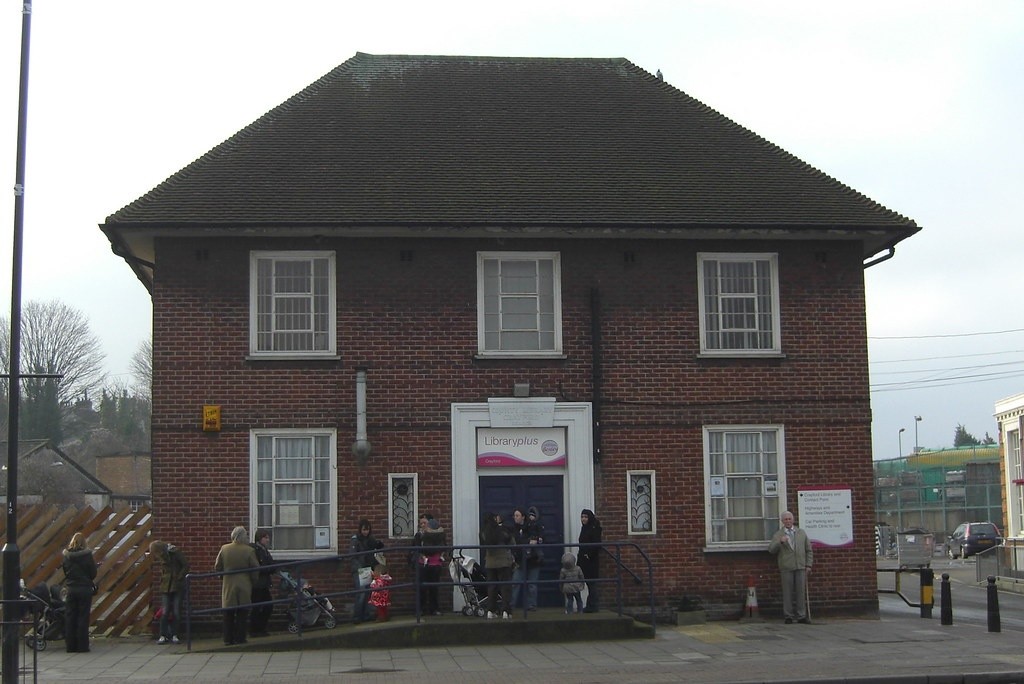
[947,521,1003,560]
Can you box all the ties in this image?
[788,528,793,546]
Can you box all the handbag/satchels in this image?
[358,567,372,587]
[565,583,588,612]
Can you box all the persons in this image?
[150,540,191,644]
[368,565,393,622]
[347,518,384,625]
[246,528,279,638]
[215,526,261,646]
[407,515,448,617]
[576,509,602,613]
[508,505,545,612]
[479,509,516,620]
[768,511,813,625]
[61,533,97,652]
[559,553,586,615]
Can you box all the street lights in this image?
[899,428,905,485]
[915,416,922,508]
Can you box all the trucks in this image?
[896,528,933,569]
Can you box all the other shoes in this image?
[502,611,512,619]
[173,635,179,642]
[784,619,793,624]
[159,635,166,642]
[798,617,809,623]
[488,611,498,619]
[440,556,445,562]
[424,558,428,567]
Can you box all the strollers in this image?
[449,548,512,617]
[19,577,67,651]
[274,568,336,634]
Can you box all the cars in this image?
[92,513,153,588]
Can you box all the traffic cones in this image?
[740,575,766,623]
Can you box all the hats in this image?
[427,519,438,529]
